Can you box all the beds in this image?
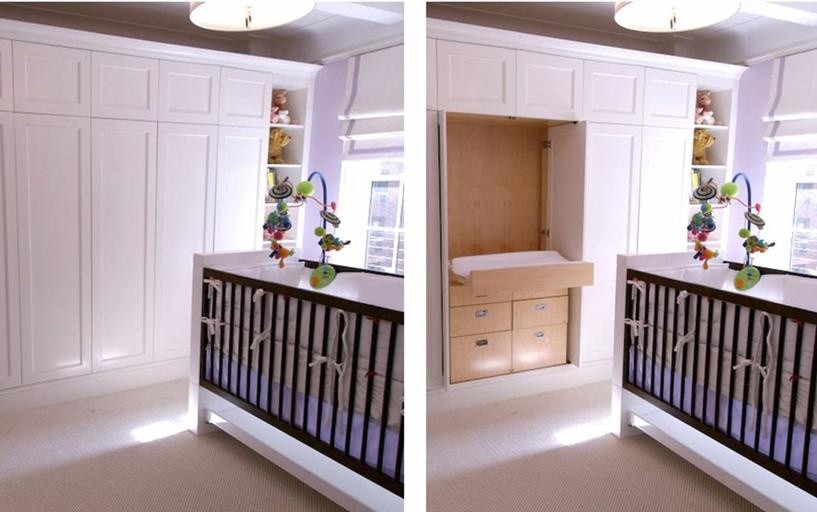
[194,246,403,512]
[605,244,816,512]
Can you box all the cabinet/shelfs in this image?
[427,19,745,402]
[0,19,325,397]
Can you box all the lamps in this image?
[188,2,316,31]
[616,0,743,35]
